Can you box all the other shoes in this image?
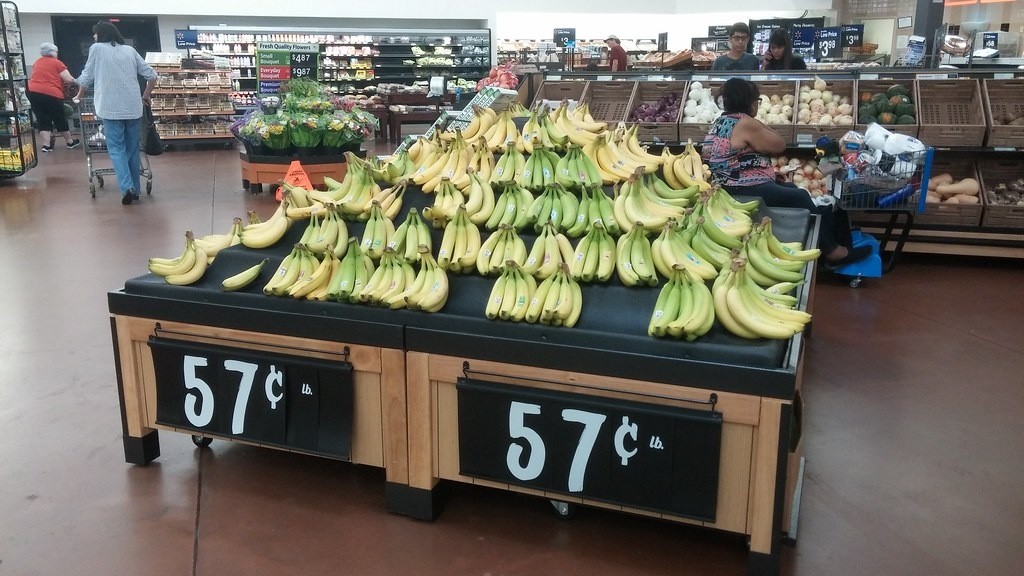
[134,196,139,200]
[825,244,873,269]
[122,188,134,205]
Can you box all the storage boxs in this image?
[527,75,1024,232]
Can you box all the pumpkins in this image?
[858,85,916,125]
[915,172,979,205]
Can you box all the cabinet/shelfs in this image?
[147,67,236,138]
[190,30,493,113]
[0,1,38,178]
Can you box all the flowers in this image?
[229,75,380,147]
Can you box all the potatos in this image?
[987,177,1024,207]
[992,111,1024,126]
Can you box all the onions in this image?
[682,75,853,197]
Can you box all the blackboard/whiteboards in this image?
[813,27,843,58]
[747,16,825,56]
[553,29,575,47]
[175,30,198,49]
[983,33,998,49]
[792,27,814,47]
[842,24,864,46]
[709,26,731,35]
[658,32,667,52]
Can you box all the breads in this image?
[642,49,722,62]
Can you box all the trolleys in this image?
[828,146,934,275]
[74,97,153,199]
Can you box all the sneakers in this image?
[41,145,53,151]
[67,138,80,149]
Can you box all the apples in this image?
[476,67,518,90]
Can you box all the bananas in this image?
[220,98,822,342]
[148,211,261,285]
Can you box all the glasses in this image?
[753,98,762,105]
[98,20,102,27]
[732,34,749,41]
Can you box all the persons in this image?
[27,42,80,152]
[709,22,759,73]
[71,22,157,205]
[603,35,627,72]
[584,61,598,71]
[702,78,873,268]
[760,27,807,70]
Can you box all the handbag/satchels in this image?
[144,100,164,155]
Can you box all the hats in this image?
[603,34,620,44]
